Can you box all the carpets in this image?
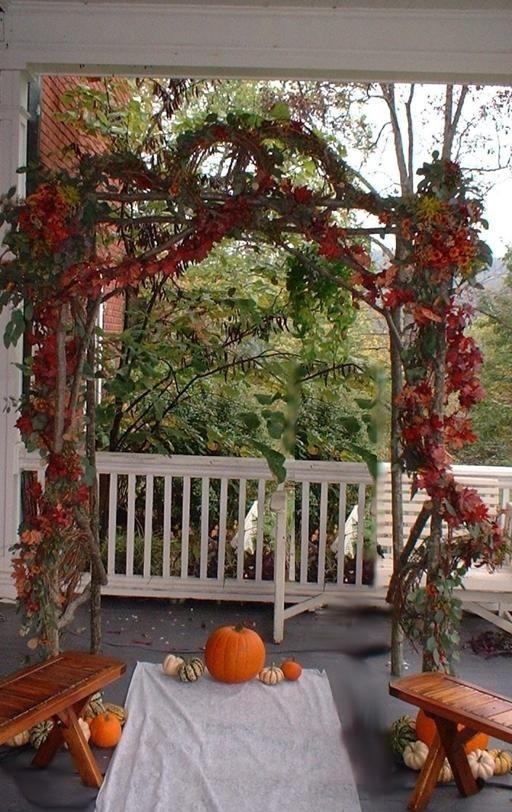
[91,660,363,811]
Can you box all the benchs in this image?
[388,672,511,809]
[2,645,129,795]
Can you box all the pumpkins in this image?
[391,708,511,783]
[5,693,129,748]
[163,623,302,685]
